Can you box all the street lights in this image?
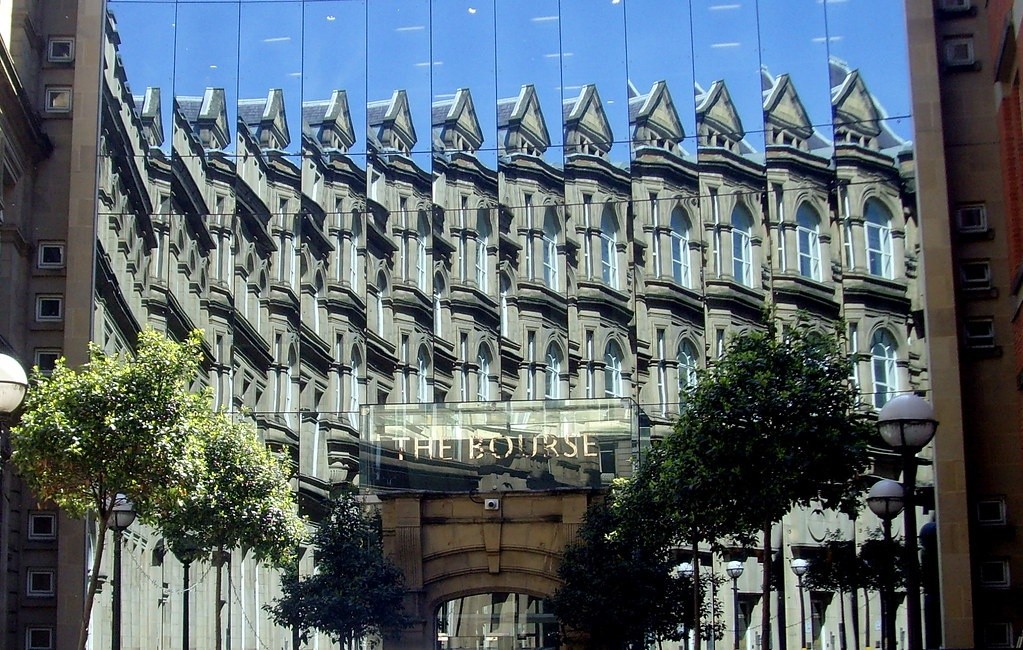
[726,560,744,650]
[867,479,907,650]
[677,562,695,650]
[874,395,940,650]
[170,534,200,650]
[790,559,809,650]
[97,493,137,650]
[0,352,30,650]
